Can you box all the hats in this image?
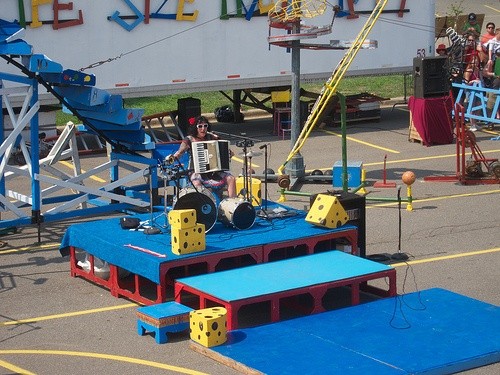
[468,12,476,22]
[435,44,446,54]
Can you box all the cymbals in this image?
[236,148,264,158]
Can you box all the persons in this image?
[439,12,500,133]
[169,116,237,197]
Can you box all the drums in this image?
[218,198,256,230]
[171,187,217,234]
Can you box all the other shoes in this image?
[469,124,482,131]
[489,124,494,130]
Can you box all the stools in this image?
[332,161,363,188]
[280,128,292,140]
[137,301,196,345]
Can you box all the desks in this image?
[174,250,397,332]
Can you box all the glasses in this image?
[196,124,208,129]
[487,27,495,30]
[466,39,474,42]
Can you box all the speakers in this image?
[310,190,365,259]
[178,97,201,141]
[413,56,450,98]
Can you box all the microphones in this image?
[205,130,219,138]
[259,144,269,149]
[397,185,401,200]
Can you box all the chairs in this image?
[271,90,291,137]
